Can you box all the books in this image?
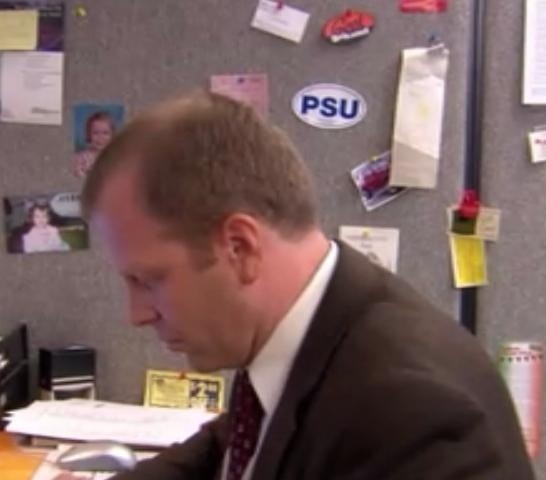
[31,436,69,447]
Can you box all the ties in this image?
[224,370,265,480]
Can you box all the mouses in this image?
[59,440,137,472]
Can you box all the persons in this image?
[75,112,115,178]
[80,93,535,480]
[22,204,70,253]
